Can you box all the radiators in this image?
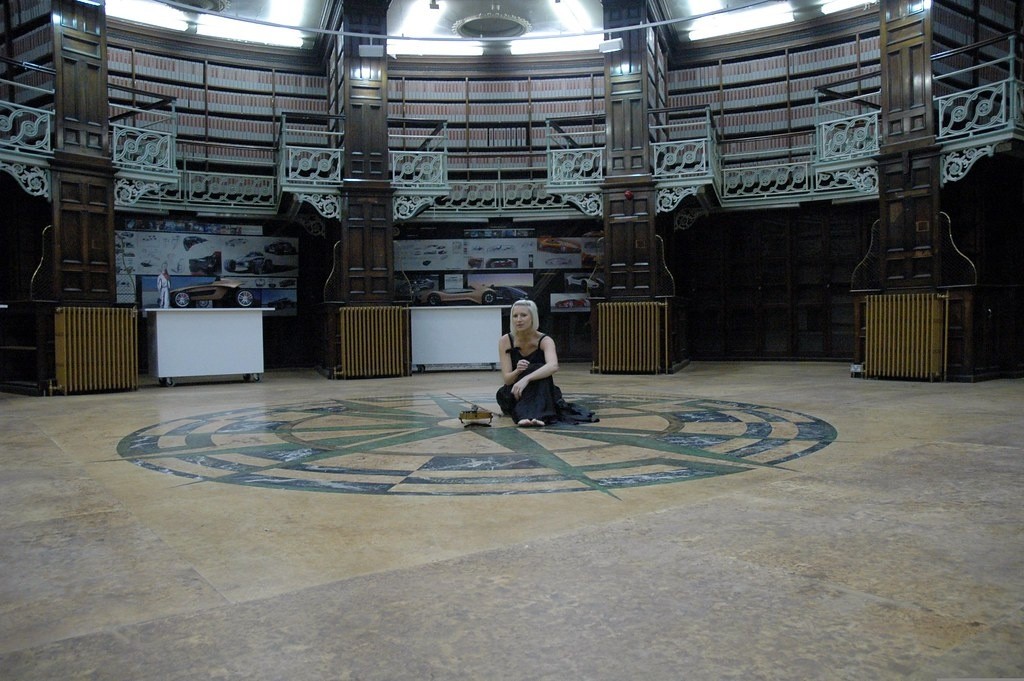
[859,291,950,381]
[331,306,411,378]
[46,306,139,396]
[592,299,669,377]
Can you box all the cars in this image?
[158,277,254,308]
[555,299,588,308]
[566,274,605,289]
[538,238,580,254]
[415,283,497,306]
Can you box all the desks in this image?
[408,305,512,372]
[140,308,276,386]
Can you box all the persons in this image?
[496,299,558,426]
[157,268,171,308]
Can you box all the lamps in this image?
[598,33,623,52]
[358,38,384,57]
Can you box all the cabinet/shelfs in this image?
[0,0,1024,199]
